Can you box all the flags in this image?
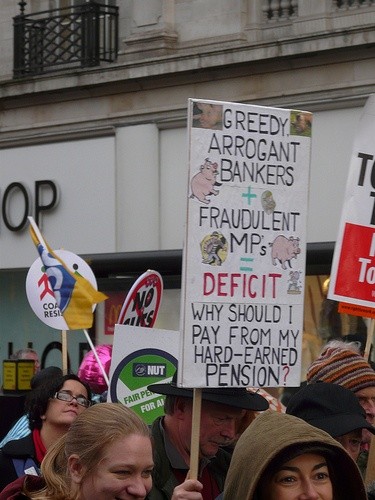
[26,215,108,330]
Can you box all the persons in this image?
[222,408,365,500]
[24,402,202,500]
[0,341,375,500]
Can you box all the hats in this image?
[286,380,375,439]
[262,441,337,483]
[31,366,63,390]
[148,368,269,411]
[306,340,375,393]
[239,387,287,433]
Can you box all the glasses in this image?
[53,392,92,408]
